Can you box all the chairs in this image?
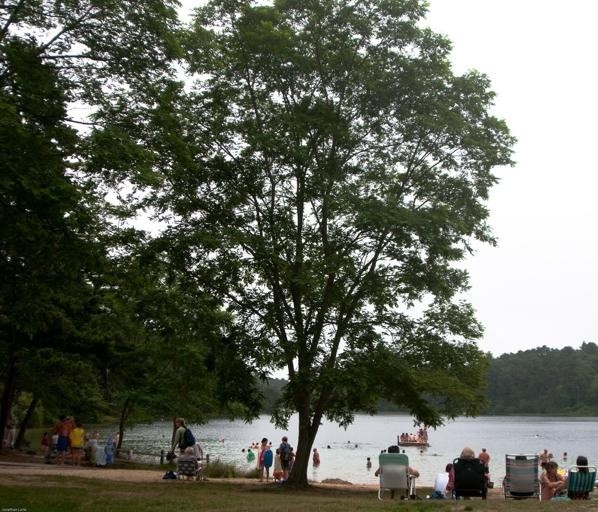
[377,453,416,501]
[452,459,488,501]
[503,455,543,504]
[568,467,596,500]
[176,458,201,482]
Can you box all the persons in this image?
[367,428,428,500]
[40,414,120,469]
[327,440,358,449]
[501,449,589,501]
[239,436,296,485]
[430,447,490,499]
[3,413,17,449]
[171,416,209,481]
[313,448,320,466]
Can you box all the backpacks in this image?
[183,429,195,446]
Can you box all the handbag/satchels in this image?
[431,490,442,498]
[163,472,177,479]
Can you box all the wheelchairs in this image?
[177,456,203,480]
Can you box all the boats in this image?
[397,435,429,447]
